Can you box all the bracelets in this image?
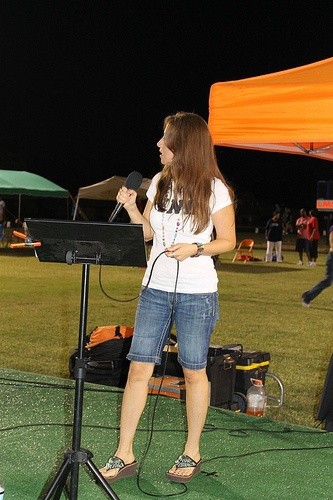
[191,243,204,258]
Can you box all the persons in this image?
[296,208,319,266]
[265,210,283,263]
[302,225,333,307]
[100,112,236,483]
[273,204,293,234]
[0,200,5,240]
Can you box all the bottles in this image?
[247,378,265,416]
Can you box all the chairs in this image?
[232,238,254,263]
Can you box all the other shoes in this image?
[298,260,304,265]
[307,261,316,266]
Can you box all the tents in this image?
[73,176,153,221]
[209,56,333,160]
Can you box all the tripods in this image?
[23,217,148,500]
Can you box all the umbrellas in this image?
[0,169,70,243]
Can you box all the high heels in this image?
[169,453,203,483]
[99,455,138,481]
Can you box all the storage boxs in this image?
[237,351,271,400]
[152,350,234,407]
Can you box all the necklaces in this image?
[161,207,179,247]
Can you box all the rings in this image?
[171,252,175,258]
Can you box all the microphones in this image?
[108,172,142,223]
